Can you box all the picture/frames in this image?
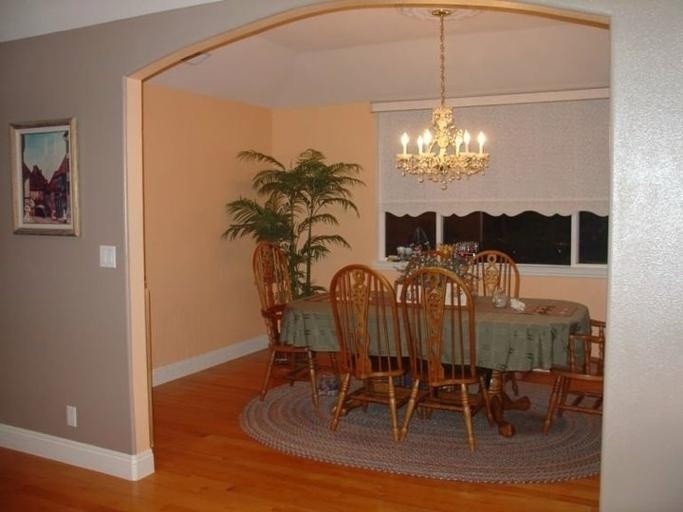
[8,116,81,238]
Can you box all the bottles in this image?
[388,242,478,266]
[491,287,507,307]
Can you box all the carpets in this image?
[238,375,601,479]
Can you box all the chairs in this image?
[542,318,606,434]
[251,239,340,407]
[460,251,521,400]
[326,263,428,440]
[399,267,495,449]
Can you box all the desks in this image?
[276,290,592,414]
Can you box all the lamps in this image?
[393,10,490,189]
